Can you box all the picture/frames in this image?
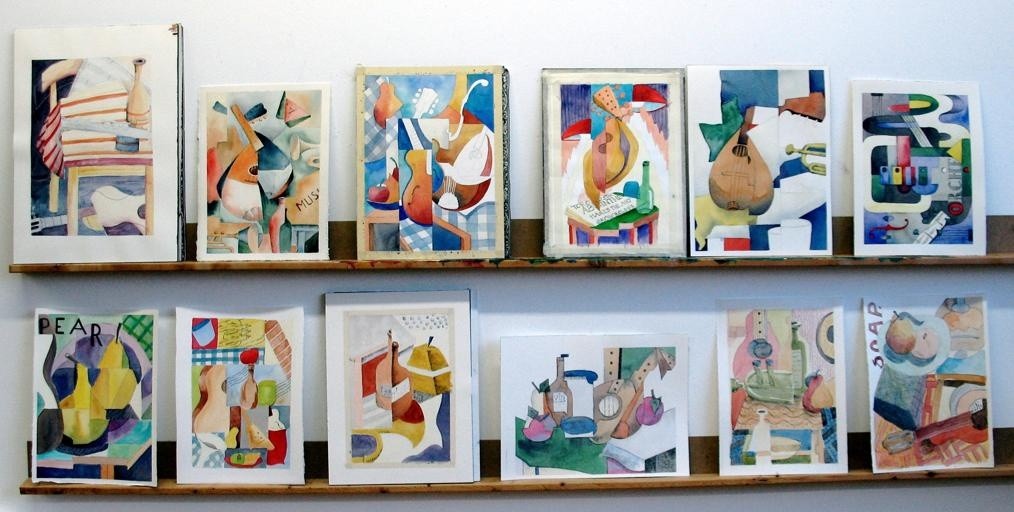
[317,280,483,490]
[845,76,996,262]
[489,326,697,487]
[542,62,688,262]
[192,80,336,268]
[685,59,836,262]
[172,296,313,491]
[861,287,996,480]
[354,60,518,264]
[716,291,853,485]
[16,302,162,493]
[9,21,192,264]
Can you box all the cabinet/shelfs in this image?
[16,202,1014,496]
